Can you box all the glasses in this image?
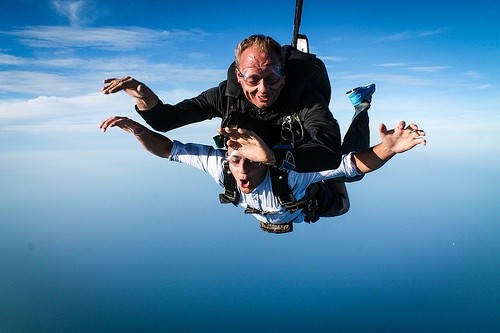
[224,152,268,172]
[238,61,281,90]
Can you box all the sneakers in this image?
[348,83,381,108]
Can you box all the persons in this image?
[100,34,342,173]
[99,84,427,234]
